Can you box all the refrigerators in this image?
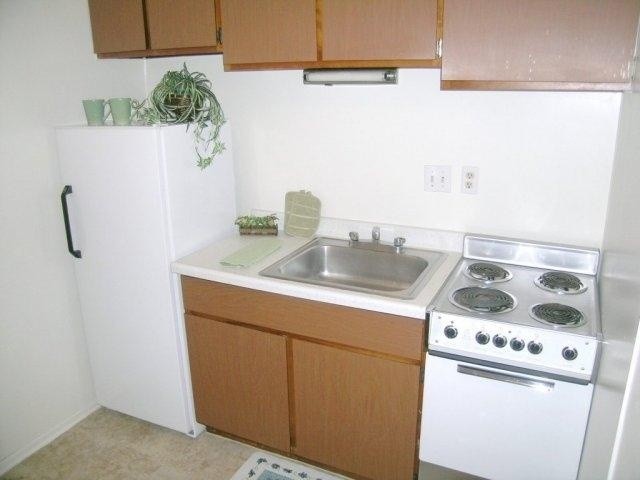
[54,115,244,439]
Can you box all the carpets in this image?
[229,451,349,480]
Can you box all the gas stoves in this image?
[427,260,605,379]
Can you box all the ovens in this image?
[417,355,595,479]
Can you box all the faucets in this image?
[258,235,448,301]
[371,225,381,249]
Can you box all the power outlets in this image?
[424,165,450,191]
[461,166,478,193]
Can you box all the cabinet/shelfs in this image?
[221,0,443,70]
[87,0,222,59]
[180,274,426,480]
[441,0,640,91]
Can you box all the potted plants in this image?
[137,62,226,169]
[234,213,279,235]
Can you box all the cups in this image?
[107,97,141,127]
[81,98,111,127]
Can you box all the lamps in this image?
[302,68,397,84]
